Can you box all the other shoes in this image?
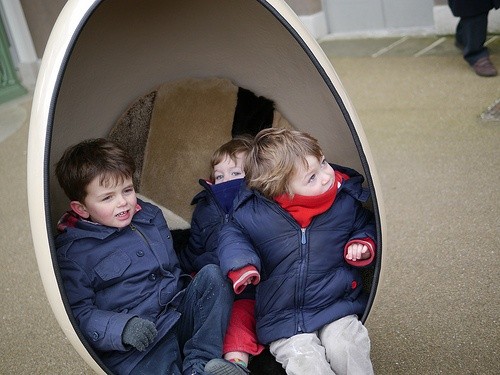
[473,55,497,76]
[203,358,251,375]
[453,41,466,51]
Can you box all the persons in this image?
[53,138,234,375]
[179,135,266,375]
[218,128,377,375]
[448,0,500,76]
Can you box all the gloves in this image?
[122,316,158,351]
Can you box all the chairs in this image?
[26,0,386,375]
